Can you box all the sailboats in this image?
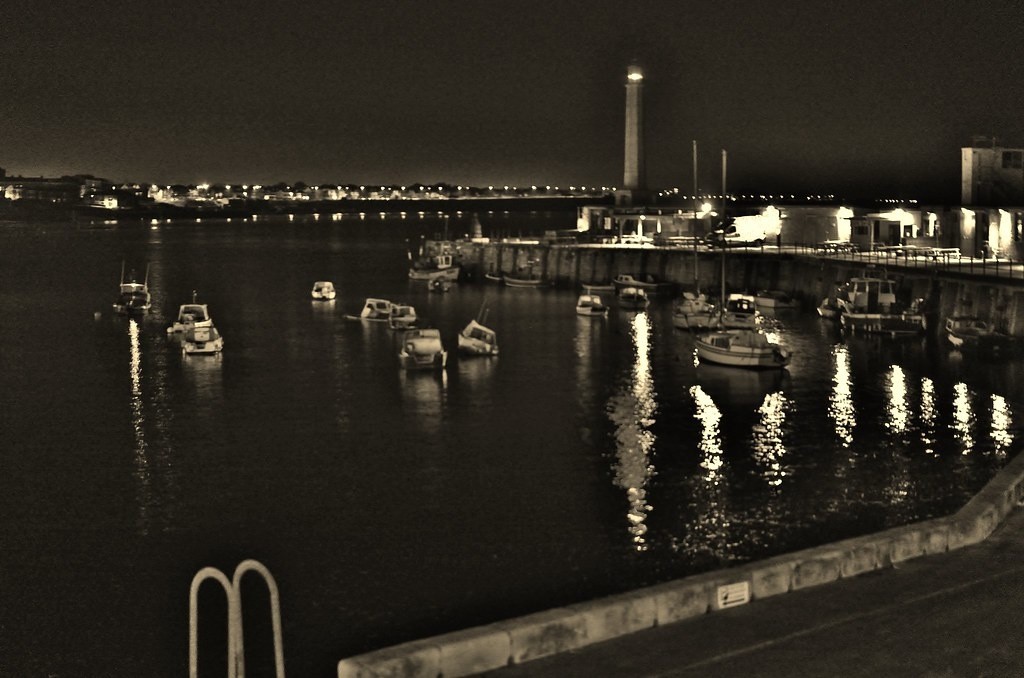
[720,150,764,328]
[671,140,722,329]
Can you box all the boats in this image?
[457,296,500,355]
[944,298,1017,362]
[583,283,616,291]
[618,287,651,311]
[347,298,417,329]
[485,271,544,288]
[755,290,800,308]
[576,295,610,316]
[398,326,448,368]
[428,276,449,292]
[613,275,658,291]
[113,260,152,315]
[166,290,224,356]
[837,264,928,335]
[312,282,336,299]
[695,329,794,367]
[817,298,837,318]
[408,254,461,281]
